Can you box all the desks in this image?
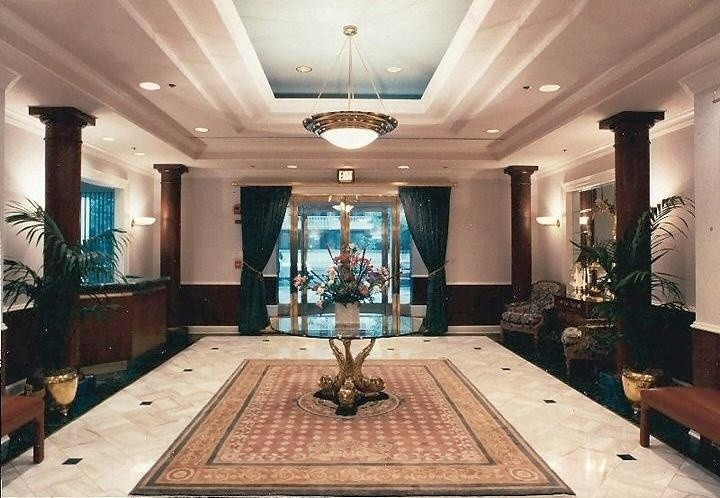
[564,290,615,320]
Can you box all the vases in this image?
[335,302,359,325]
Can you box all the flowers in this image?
[293,243,403,310]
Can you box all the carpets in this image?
[129,358,575,494]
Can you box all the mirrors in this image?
[567,192,618,289]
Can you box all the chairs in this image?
[500,280,566,350]
[560,317,618,384]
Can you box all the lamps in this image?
[303,25,398,150]
[131,217,156,226]
[536,216,560,227]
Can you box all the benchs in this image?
[0,394,45,463]
[640,386,720,447]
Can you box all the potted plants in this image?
[568,195,695,403]
[3,195,136,416]
